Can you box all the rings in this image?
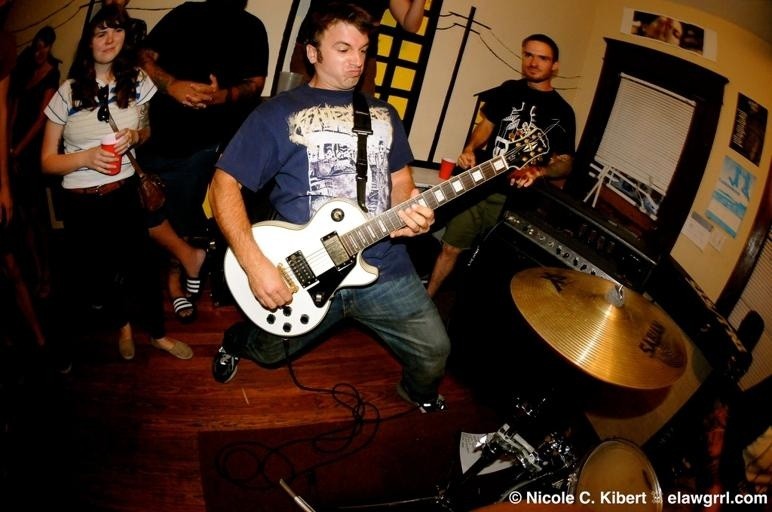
[127,142,131,148]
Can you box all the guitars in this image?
[224,131,552,337]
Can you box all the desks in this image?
[411,166,440,190]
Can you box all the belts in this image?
[68,180,124,198]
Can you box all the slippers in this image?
[168,253,212,323]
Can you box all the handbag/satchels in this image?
[138,171,166,211]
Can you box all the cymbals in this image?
[512,265,691,390]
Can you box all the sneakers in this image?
[213,346,242,385]
[395,380,448,415]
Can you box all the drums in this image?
[574,438,665,511]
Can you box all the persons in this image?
[290,0,426,97]
[102,0,147,47]
[39,3,194,360]
[0,0,73,374]
[139,0,269,325]
[207,2,452,415]
[9,26,60,299]
[427,35,576,297]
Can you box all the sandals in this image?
[114,332,194,362]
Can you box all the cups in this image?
[438,157,455,181]
[100,134,123,177]
